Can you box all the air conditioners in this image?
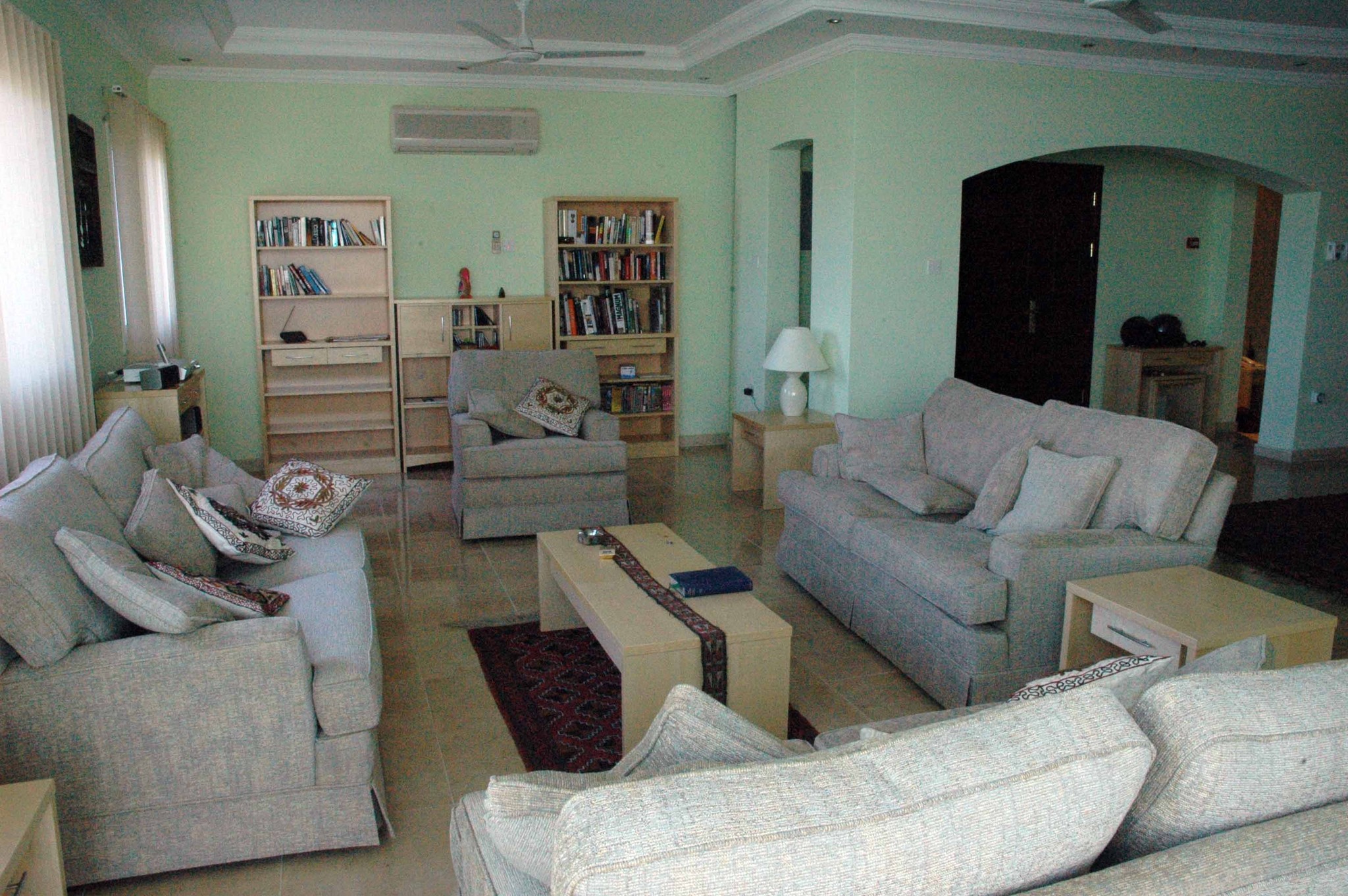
[390,102,541,153]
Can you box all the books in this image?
[558,208,673,413]
[669,566,753,599]
[256,216,385,297]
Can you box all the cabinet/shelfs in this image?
[547,198,682,459]
[392,297,561,467]
[249,199,400,478]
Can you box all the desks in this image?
[93,369,211,447]
[1239,365,1265,414]
[1104,345,1221,443]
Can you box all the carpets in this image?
[469,622,819,778]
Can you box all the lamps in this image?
[761,331,830,416]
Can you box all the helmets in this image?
[1120,316,1153,348]
[1149,314,1186,349]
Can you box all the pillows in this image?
[466,377,592,438]
[54,434,376,634]
[987,445,1120,528]
[952,435,1039,531]
[858,468,976,518]
[837,413,928,481]
[611,637,1277,783]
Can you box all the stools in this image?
[1142,374,1206,428]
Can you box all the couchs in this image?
[1,408,397,889]
[449,346,630,542]
[775,378,1236,708]
[448,644,1348,896]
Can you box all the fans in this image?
[456,0,645,73]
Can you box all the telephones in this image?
[1241,356,1255,367]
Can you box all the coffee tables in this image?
[538,528,795,762]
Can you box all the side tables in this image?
[1,778,68,896]
[1058,570,1338,689]
[728,413,835,511]
